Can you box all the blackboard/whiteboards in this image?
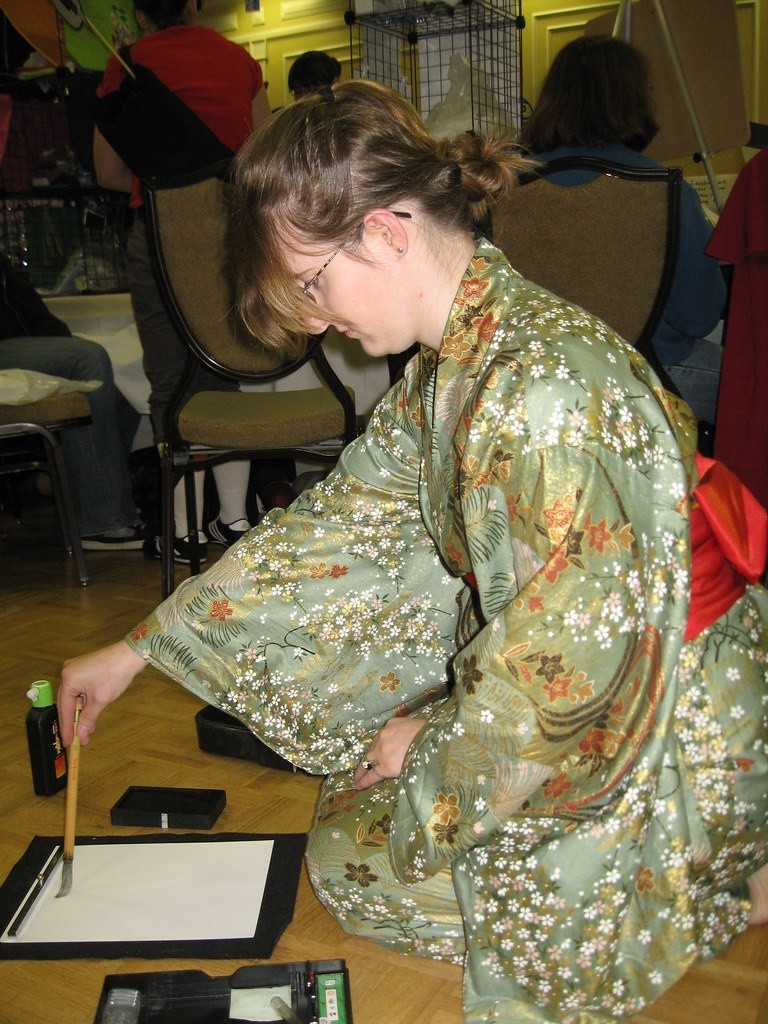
[583,0,752,163]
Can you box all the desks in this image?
[38,293,153,416]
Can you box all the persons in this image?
[57,51,768,1024]
[0,0,730,565]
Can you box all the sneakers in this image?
[79,520,152,551]
[205,515,252,549]
[142,530,208,563]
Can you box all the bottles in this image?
[26,680,68,797]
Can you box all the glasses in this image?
[296,211,412,303]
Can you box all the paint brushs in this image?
[54,696,84,899]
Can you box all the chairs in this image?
[141,156,365,600]
[0,392,94,587]
[481,161,715,445]
